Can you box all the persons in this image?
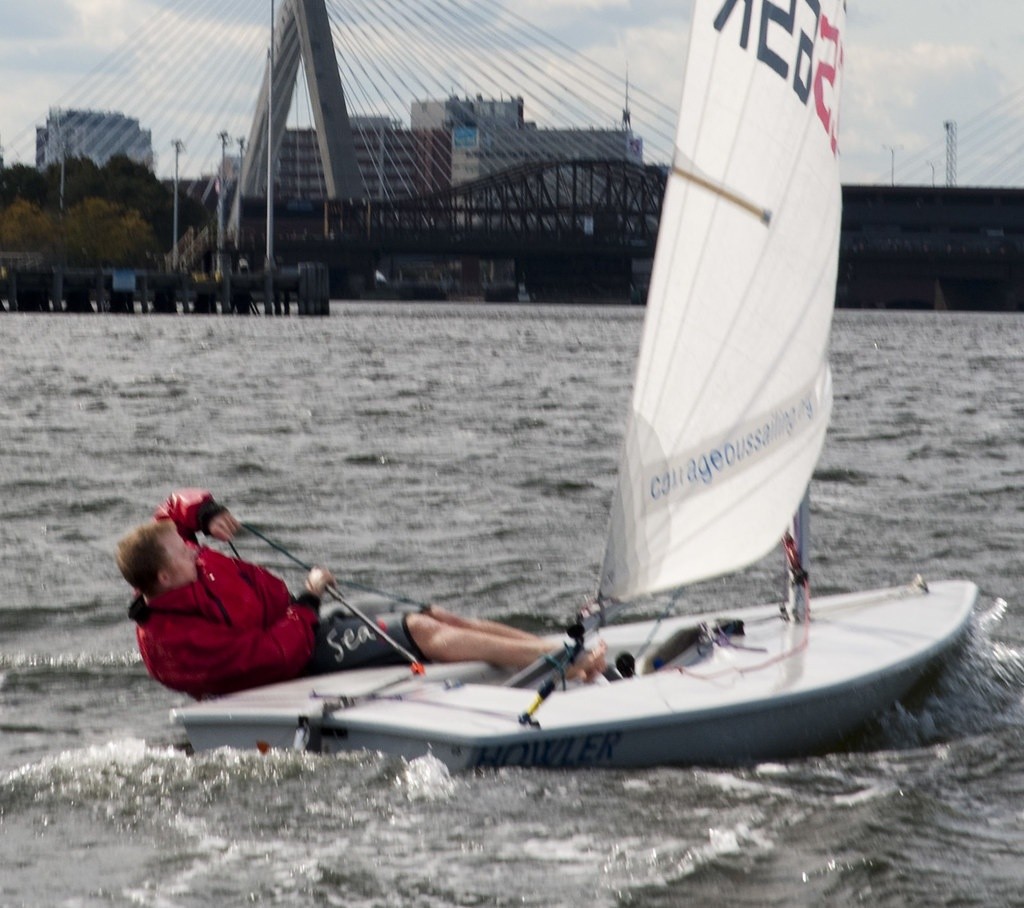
[120,487,607,704]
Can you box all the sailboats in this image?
[167,0,985,781]
